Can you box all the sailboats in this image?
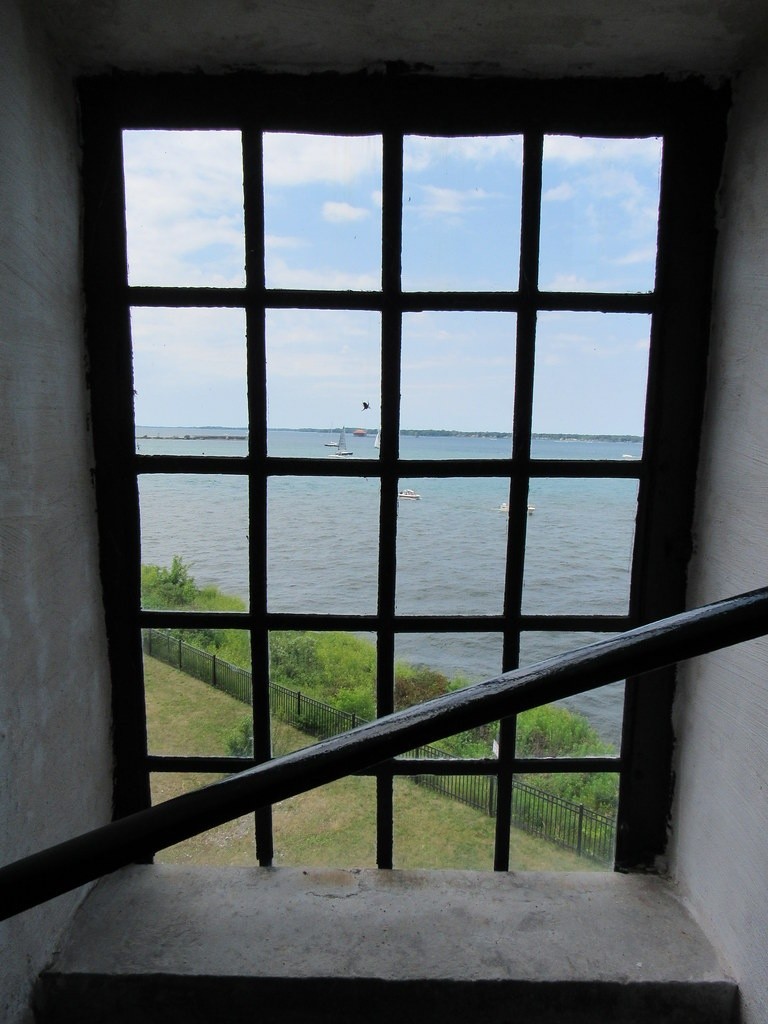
[325,423,338,447]
[335,426,353,455]
[373,425,380,449]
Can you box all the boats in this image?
[398,489,420,499]
[499,502,535,513]
[623,454,635,458]
